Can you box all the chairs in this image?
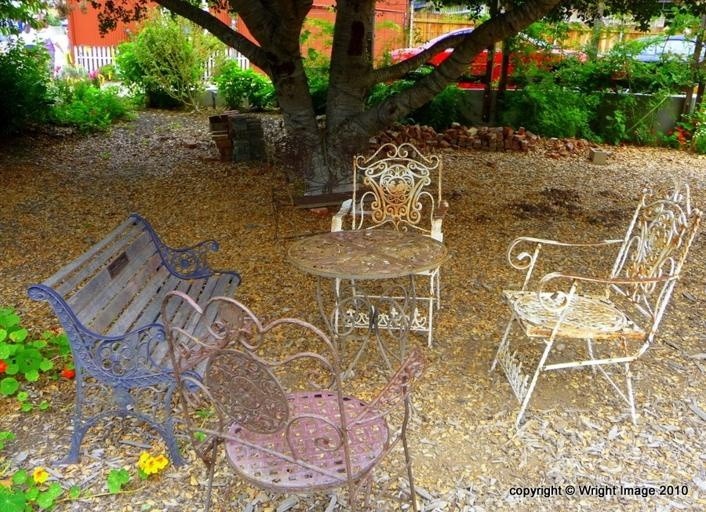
[489,184,704,428]
[331,143,449,346]
[161,290,431,511]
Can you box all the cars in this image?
[610,34,705,65]
[379,28,627,90]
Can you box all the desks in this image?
[287,229,453,391]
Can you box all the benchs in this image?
[269,185,383,241]
[27,211,245,470]
